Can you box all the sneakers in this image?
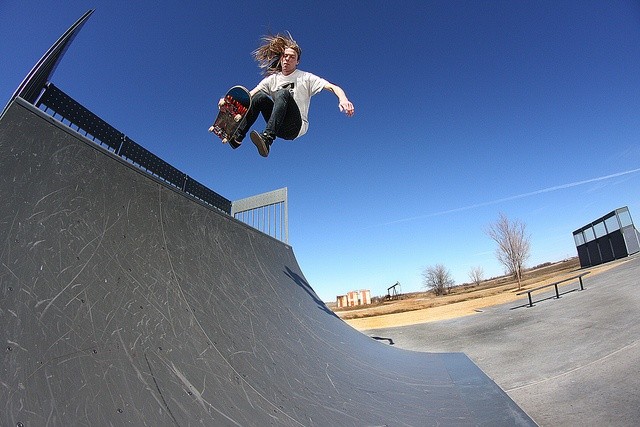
[229,138,242,149]
[250,130,269,157]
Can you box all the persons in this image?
[217,30,355,158]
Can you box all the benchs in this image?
[516,270,591,309]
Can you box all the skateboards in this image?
[208,85,252,145]
